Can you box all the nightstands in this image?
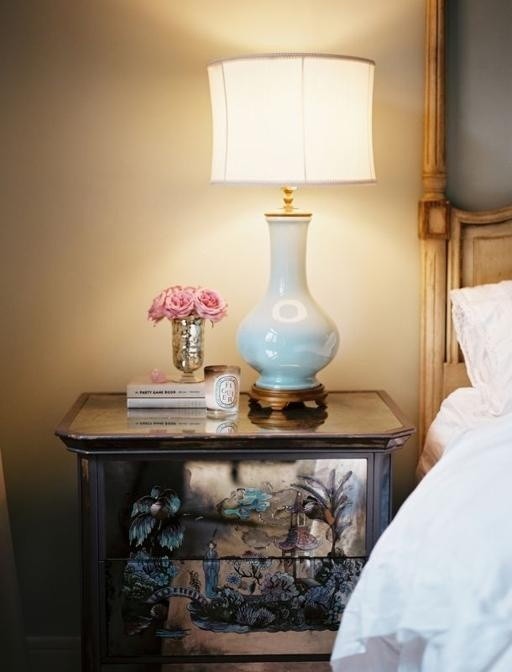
[56,389,416,672]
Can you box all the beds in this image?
[329,0,511,672]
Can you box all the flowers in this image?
[147,286,229,327]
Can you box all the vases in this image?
[171,317,204,384]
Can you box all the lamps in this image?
[208,52,377,413]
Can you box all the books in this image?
[126,382,206,398]
[127,410,208,434]
[126,398,206,409]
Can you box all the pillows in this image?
[448,278,512,416]
